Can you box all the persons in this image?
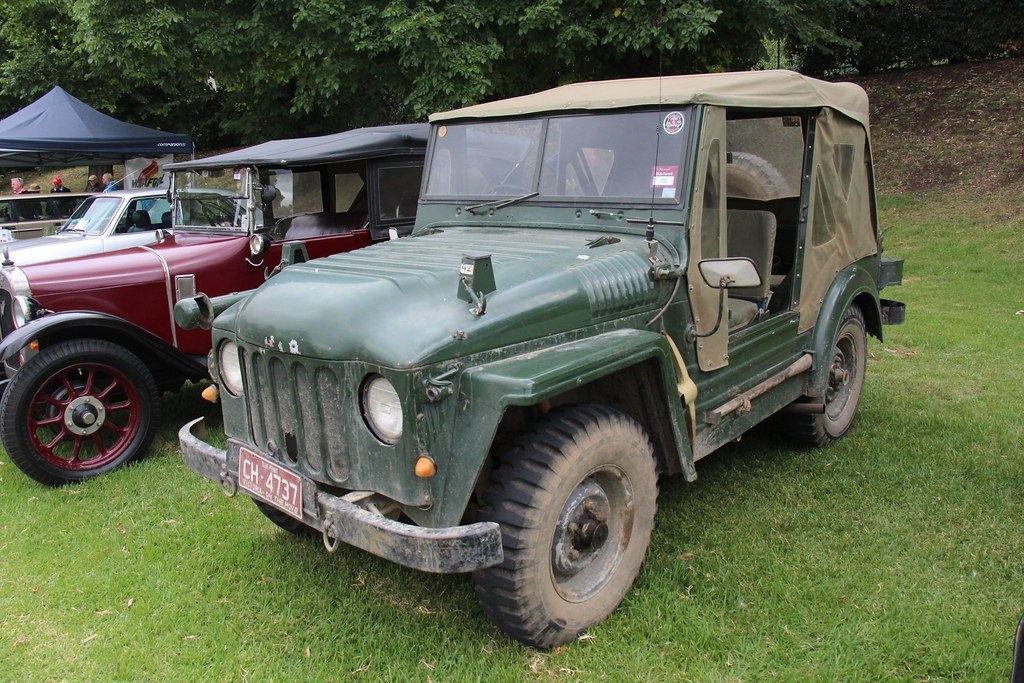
[3,170,120,223]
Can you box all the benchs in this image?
[283,212,369,239]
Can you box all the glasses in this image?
[89,180,95,182]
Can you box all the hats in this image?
[52,178,62,184]
[27,183,40,191]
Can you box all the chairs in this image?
[161,212,172,229]
[126,210,153,232]
[728,209,776,333]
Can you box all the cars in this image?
[0,187,266,258]
[0,191,103,244]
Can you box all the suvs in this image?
[0,122,521,489]
[178,66,908,659]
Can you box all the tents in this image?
[0,87,194,199]
